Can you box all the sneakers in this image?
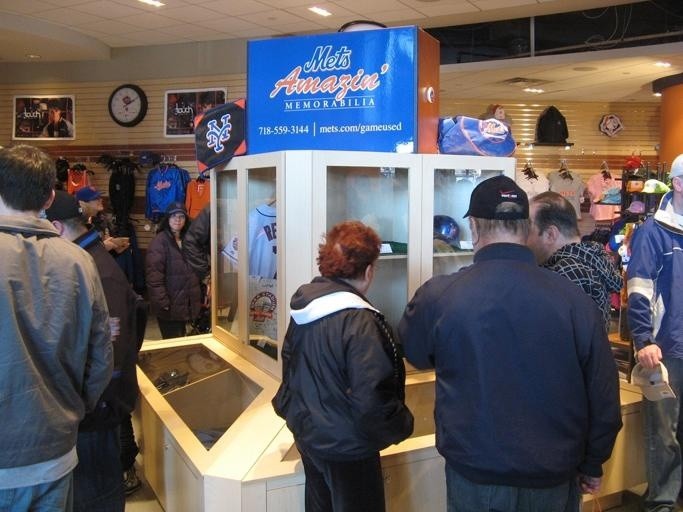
[123,476,142,494]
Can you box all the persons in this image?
[272,220,413,512]
[77,185,133,253]
[16,98,74,138]
[396,175,624,512]
[44,189,137,511]
[181,201,211,302]
[168,93,218,131]
[625,153,683,512]
[0,144,115,512]
[118,263,147,496]
[608,200,645,236]
[146,202,202,340]
[526,191,624,340]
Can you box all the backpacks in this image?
[536,105,568,142]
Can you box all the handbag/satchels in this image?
[627,181,642,192]
[624,149,642,169]
[438,115,516,157]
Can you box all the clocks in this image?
[108,84,148,127]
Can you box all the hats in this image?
[668,153,682,180]
[630,360,676,402]
[626,201,645,214]
[618,245,630,263]
[464,175,529,220]
[641,178,657,193]
[167,202,186,213]
[75,187,106,202]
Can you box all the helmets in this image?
[433,215,461,250]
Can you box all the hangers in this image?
[70,158,95,176]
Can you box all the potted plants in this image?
[95,152,144,220]
